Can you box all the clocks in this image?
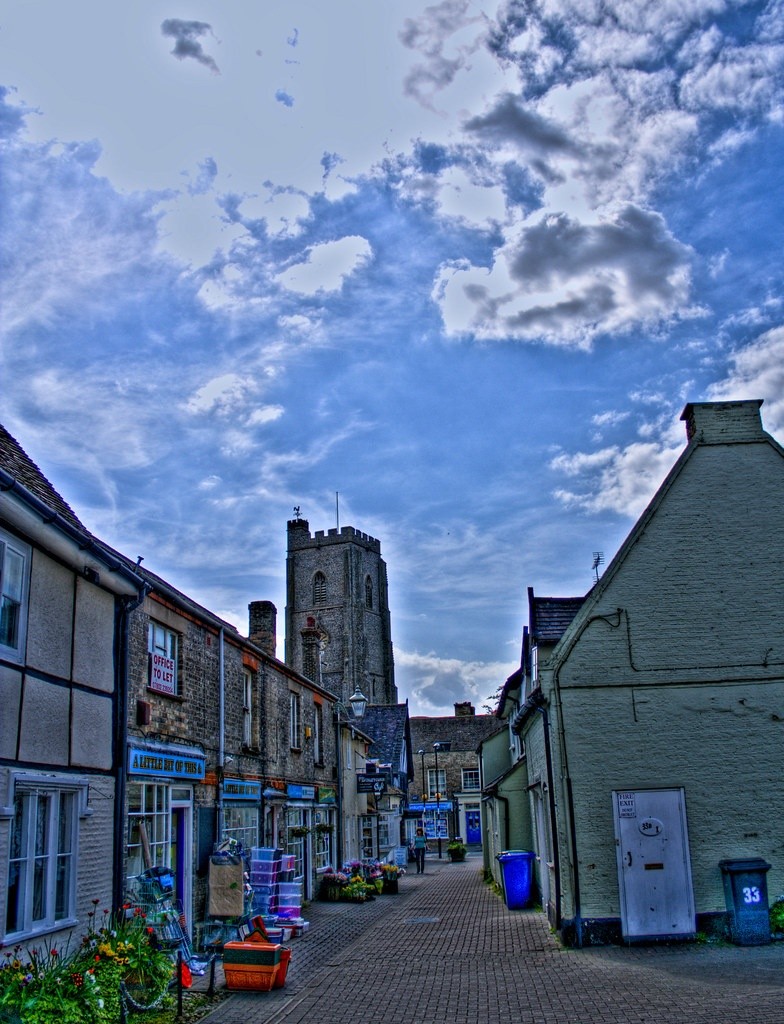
[318,632,329,651]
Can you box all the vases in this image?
[344,894,365,900]
[327,886,340,901]
[383,880,398,894]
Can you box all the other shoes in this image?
[417,872,419,874]
[421,870,424,873]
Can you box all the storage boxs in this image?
[208,846,310,991]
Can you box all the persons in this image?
[410,828,431,875]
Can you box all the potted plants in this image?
[447,842,467,862]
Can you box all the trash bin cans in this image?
[495,849,537,912]
[718,857,774,948]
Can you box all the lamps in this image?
[332,684,368,726]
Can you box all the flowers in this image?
[320,863,406,893]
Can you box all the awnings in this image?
[406,801,453,813]
